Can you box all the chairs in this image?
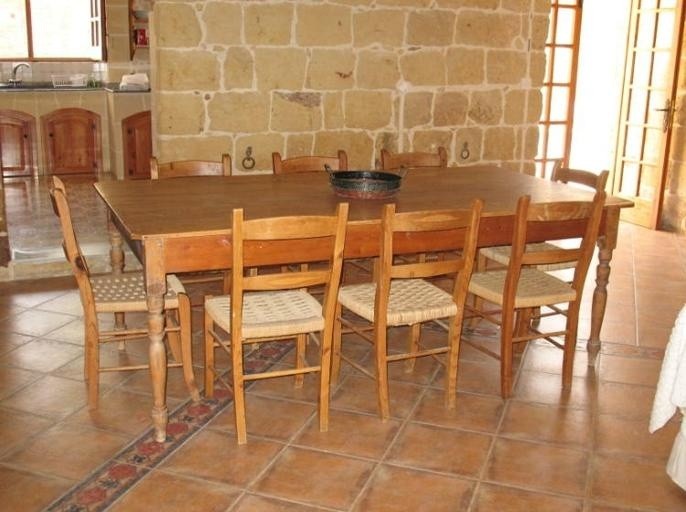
[329,197,486,424]
[205,202,349,448]
[148,154,236,294]
[455,189,607,398]
[380,147,481,330]
[273,150,348,172]
[465,159,610,353]
[46,176,194,411]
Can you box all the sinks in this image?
[0,84,54,91]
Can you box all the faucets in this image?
[9,61,31,87]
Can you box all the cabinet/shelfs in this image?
[122,111,152,179]
[129,0,151,61]
[39,107,103,175]
[0,108,38,178]
